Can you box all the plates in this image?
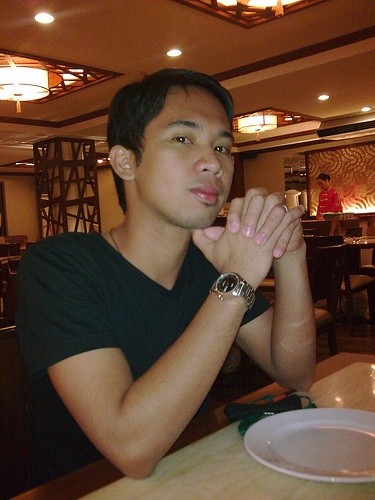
[243,408,375,484]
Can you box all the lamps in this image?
[0,67,50,113]
[238,115,278,143]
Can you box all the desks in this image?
[13,353,375,500]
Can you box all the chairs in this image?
[0,235,37,500]
[257,221,375,357]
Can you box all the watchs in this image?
[209,271,255,313]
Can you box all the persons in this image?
[14,65,317,490]
[315,173,343,220]
